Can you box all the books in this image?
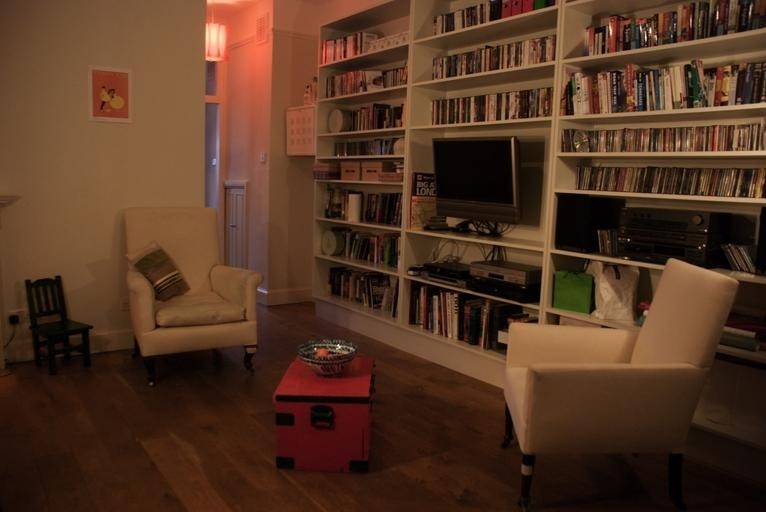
[431,35,556,80]
[365,192,402,226]
[342,232,401,270]
[430,87,552,125]
[326,190,350,221]
[560,122,766,152]
[350,102,404,131]
[560,60,766,115]
[321,32,378,65]
[409,280,538,356]
[312,162,339,180]
[326,65,408,98]
[328,267,399,318]
[378,161,403,182]
[576,166,766,198]
[596,227,619,258]
[720,242,761,275]
[334,137,405,156]
[639,305,766,352]
[432,1,556,36]
[410,172,437,231]
[583,0,766,56]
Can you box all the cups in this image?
[348,193,362,222]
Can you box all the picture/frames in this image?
[87,66,133,124]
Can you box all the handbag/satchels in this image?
[591,261,640,328]
[554,269,594,314]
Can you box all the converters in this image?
[9,314,20,327]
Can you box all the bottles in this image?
[331,188,342,217]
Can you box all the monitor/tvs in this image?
[430,134,523,227]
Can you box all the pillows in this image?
[125,239,190,303]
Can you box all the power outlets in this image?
[7,310,27,327]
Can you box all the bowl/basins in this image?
[297,339,360,375]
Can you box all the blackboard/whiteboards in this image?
[285,104,317,156]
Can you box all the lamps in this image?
[206,1,228,63]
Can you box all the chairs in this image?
[121,205,263,388]
[501,258,738,512]
[25,276,94,376]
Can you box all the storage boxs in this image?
[272,346,373,473]
